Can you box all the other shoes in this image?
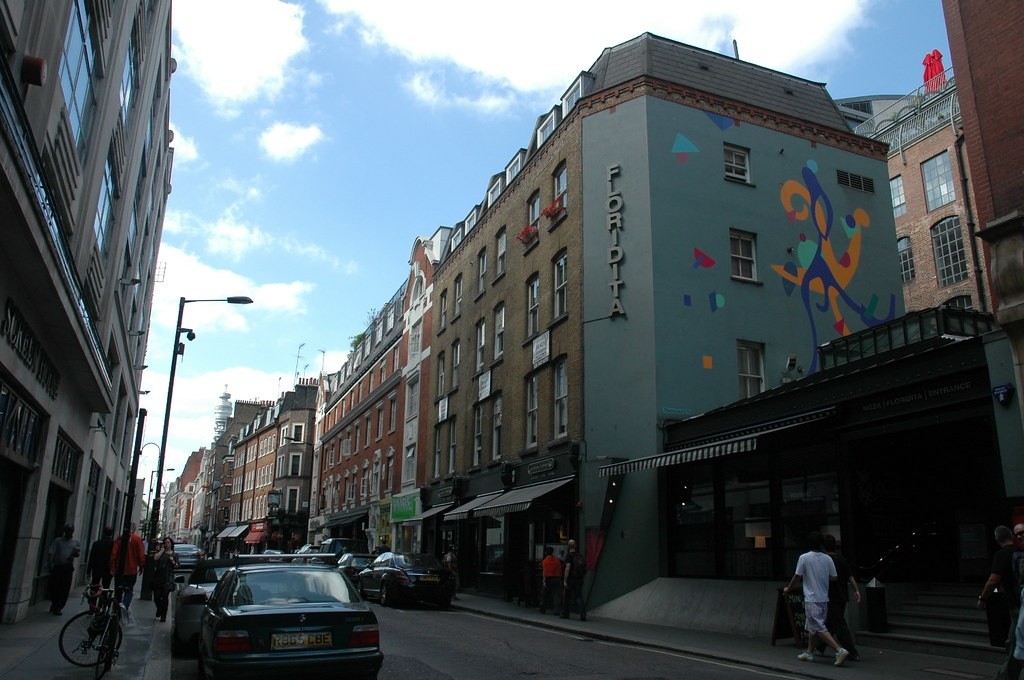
[581,615,585,621]
[53,607,62,616]
[548,608,560,616]
[156,608,166,622]
[560,613,569,619]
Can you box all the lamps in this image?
[128,330,145,337]
[784,353,797,374]
[138,390,150,395]
[122,278,141,285]
[135,365,148,371]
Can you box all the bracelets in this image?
[978,595,986,602]
[172,560,176,566]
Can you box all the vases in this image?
[550,206,560,217]
[521,232,535,245]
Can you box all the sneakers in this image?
[798,652,815,663]
[835,648,849,666]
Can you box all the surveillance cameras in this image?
[187,332,195,341]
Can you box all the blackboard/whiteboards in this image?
[770,587,807,650]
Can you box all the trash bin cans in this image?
[865,585,887,633]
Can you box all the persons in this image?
[975,523,1024,680]
[783,532,851,668]
[86,527,115,616]
[812,535,861,661]
[108,523,145,613]
[534,547,562,614]
[443,546,462,600]
[560,539,586,621]
[152,537,179,622]
[48,523,81,615]
[371,537,390,554]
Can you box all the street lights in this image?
[201,486,218,558]
[284,437,315,564]
[140,297,253,600]
[170,505,181,536]
[141,468,175,541]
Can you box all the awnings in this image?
[402,501,456,526]
[598,434,760,478]
[443,474,576,521]
[216,525,249,541]
[244,531,270,543]
[315,513,367,529]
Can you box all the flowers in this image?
[516,226,537,240]
[542,198,561,218]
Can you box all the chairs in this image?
[237,585,263,604]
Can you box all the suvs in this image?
[307,538,367,568]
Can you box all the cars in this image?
[332,554,378,599]
[291,543,319,564]
[171,541,207,609]
[355,551,456,610]
[259,550,287,564]
[170,559,287,659]
[197,563,384,680]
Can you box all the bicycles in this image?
[58,584,123,680]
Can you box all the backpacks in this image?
[568,552,587,576]
